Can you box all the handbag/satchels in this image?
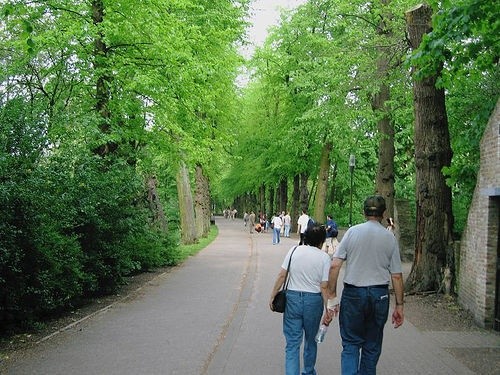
[273,290,286,313]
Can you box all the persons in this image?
[323,216,338,252]
[383,216,396,231]
[328,196,405,375]
[269,222,331,375]
[223,207,311,246]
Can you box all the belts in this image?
[344,284,388,289]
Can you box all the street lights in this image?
[350,155,355,224]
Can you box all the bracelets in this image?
[397,301,402,306]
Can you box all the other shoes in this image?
[244,209,293,246]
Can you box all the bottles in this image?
[315,323,327,343]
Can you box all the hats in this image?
[365,195,386,210]
